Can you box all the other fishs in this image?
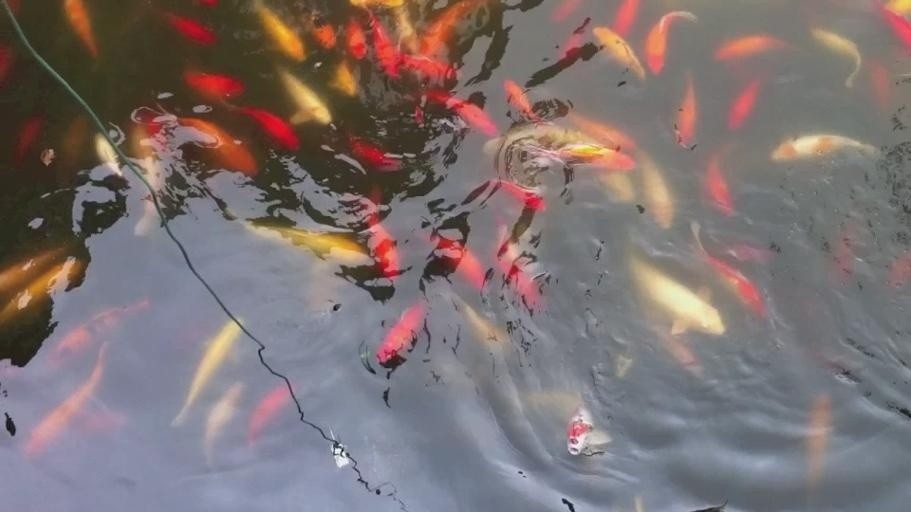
[1,0,911,376]
